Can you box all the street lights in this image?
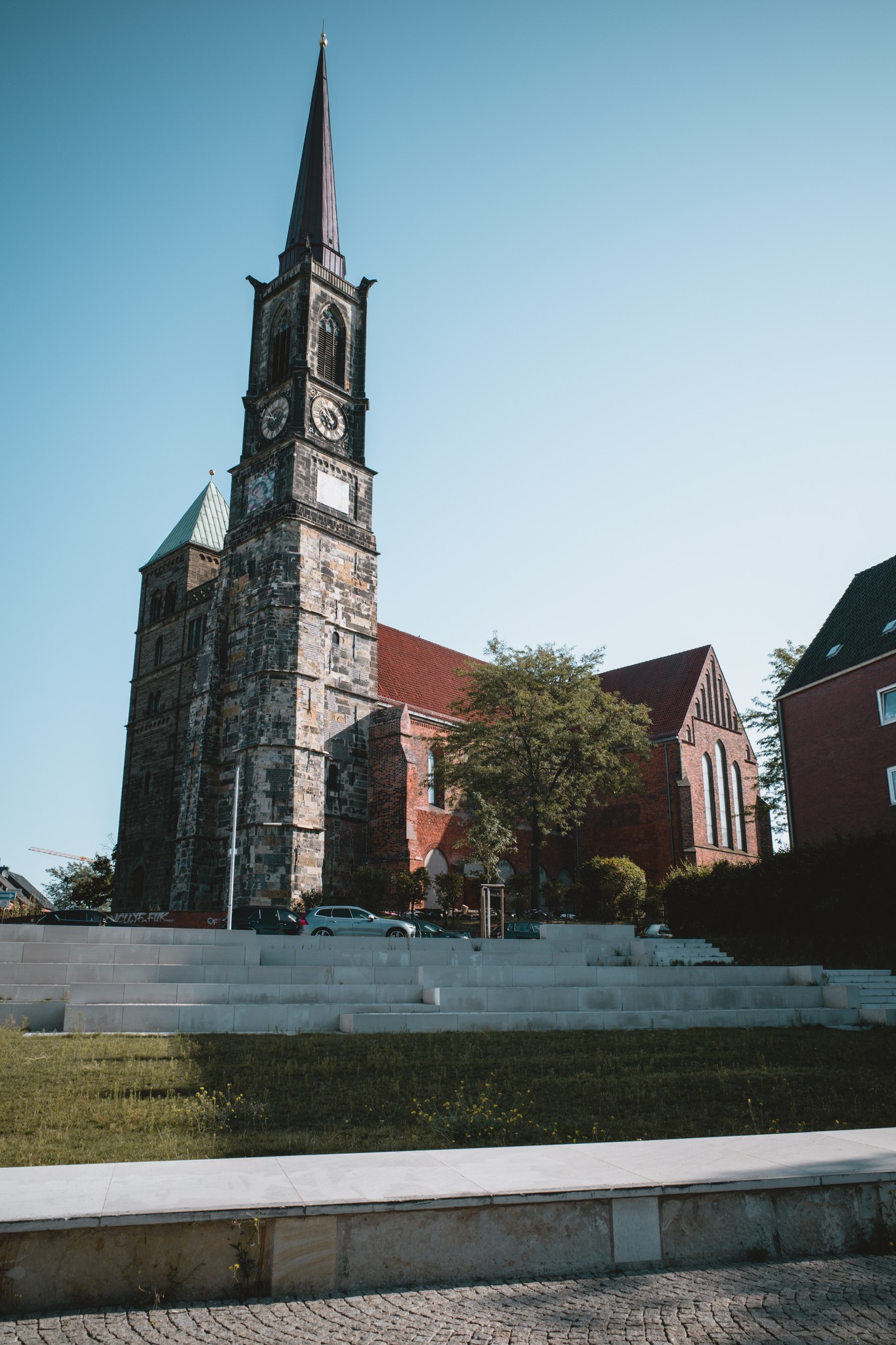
[226,767,243,929]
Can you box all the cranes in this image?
[27,845,95,864]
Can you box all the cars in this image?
[491,920,544,940]
[295,905,468,939]
[35,910,144,927]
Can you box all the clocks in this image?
[310,394,348,442]
[258,392,291,442]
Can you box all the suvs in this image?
[636,924,674,939]
[225,904,301,939]
